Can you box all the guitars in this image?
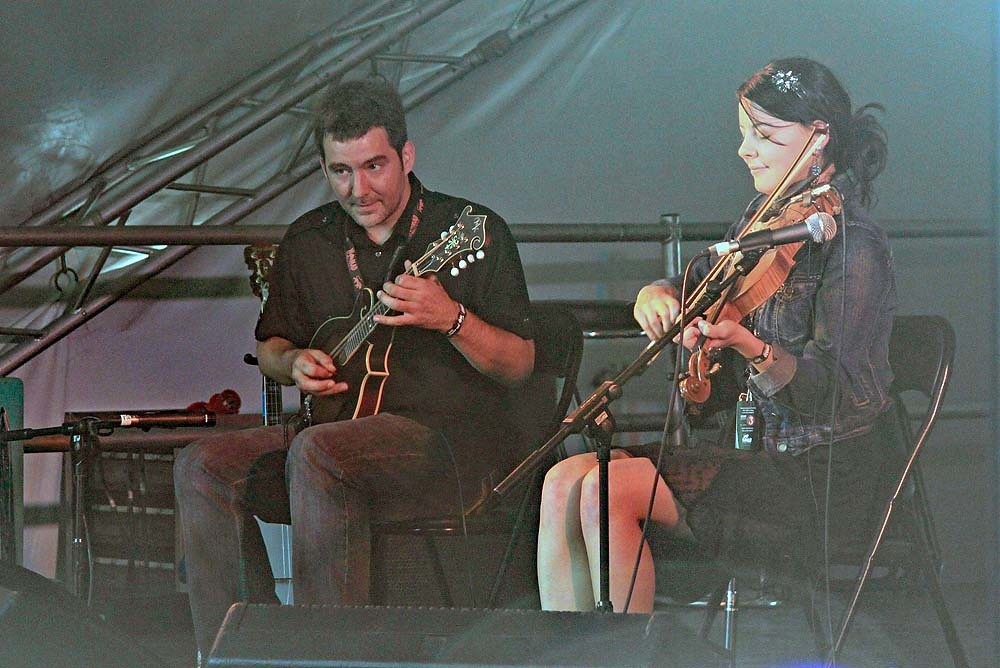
[298,203,494,429]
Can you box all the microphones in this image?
[107,410,216,427]
[701,212,836,258]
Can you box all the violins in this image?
[677,181,844,404]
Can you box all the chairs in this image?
[368,309,583,608]
[650,314,969,668]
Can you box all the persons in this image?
[535,56,899,613]
[172,78,540,668]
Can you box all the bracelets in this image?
[747,342,772,365]
[440,303,466,338]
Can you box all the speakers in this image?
[0,557,170,668]
[207,597,736,668]
